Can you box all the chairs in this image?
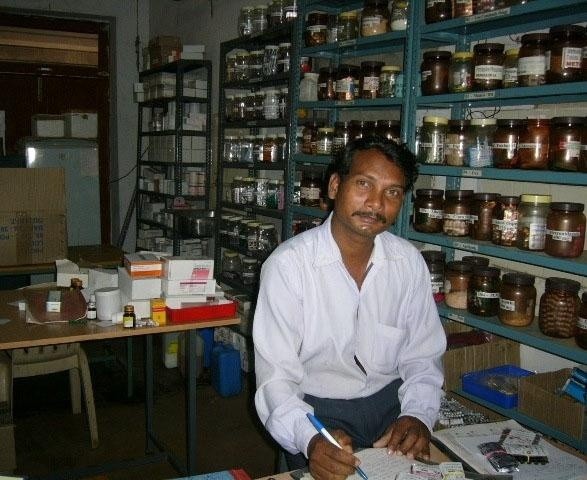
[11,282,100,452]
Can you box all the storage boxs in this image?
[53,248,238,324]
[0,167,70,265]
[140,32,180,69]
[63,108,98,141]
[30,112,64,139]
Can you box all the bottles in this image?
[111,312,124,325]
[419,1,527,23]
[154,113,162,131]
[413,251,584,348]
[87,302,96,319]
[412,113,586,177]
[410,184,584,260]
[94,286,122,320]
[416,25,586,95]
[123,305,135,328]
[220,38,289,287]
[69,277,84,294]
[292,1,408,235]
[237,1,295,39]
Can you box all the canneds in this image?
[293,1,409,237]
[220,2,299,286]
[412,2,587,350]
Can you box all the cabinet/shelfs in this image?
[131,60,213,258]
[214,0,586,460]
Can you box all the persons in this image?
[253,136,447,480]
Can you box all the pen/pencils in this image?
[306,413,368,480]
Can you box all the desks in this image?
[0,288,239,480]
[244,430,586,479]
[0,243,138,398]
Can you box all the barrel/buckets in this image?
[210,342,242,398]
[177,332,205,379]
